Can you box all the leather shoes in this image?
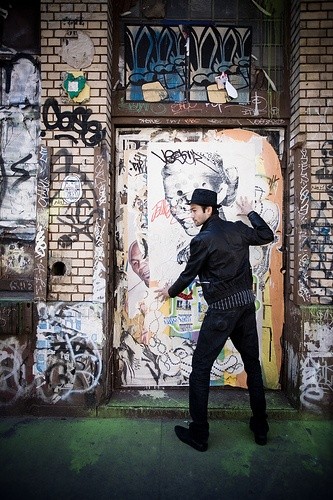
[249,415,268,445]
[174,425,209,452]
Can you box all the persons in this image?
[163,153,240,236]
[153,189,276,451]
[128,240,150,319]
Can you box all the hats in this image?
[186,189,222,208]
